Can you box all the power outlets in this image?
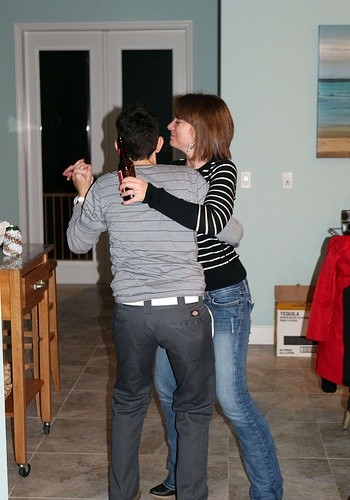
[282,173,293,189]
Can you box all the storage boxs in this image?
[274,283,321,359]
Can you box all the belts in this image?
[121,295,199,307]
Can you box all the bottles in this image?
[116,137,136,201]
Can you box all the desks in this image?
[1,242,60,479]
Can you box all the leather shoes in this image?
[149,483,176,499]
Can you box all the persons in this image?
[67,102,244,500]
[62,93,285,499]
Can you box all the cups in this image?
[3,225,23,256]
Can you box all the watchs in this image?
[73,196,85,205]
